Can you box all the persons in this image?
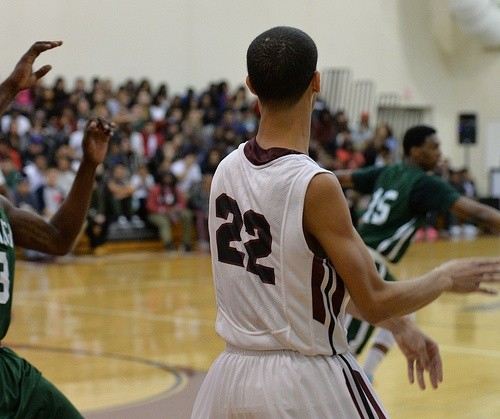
[106,164,144,231]
[131,159,154,230]
[414,210,438,241]
[190,26,500,418]
[12,178,42,224]
[330,125,500,383]
[451,168,482,239]
[34,170,66,221]
[437,159,454,187]
[147,168,195,253]
[0,41,116,419]
[1,75,395,207]
[188,176,213,250]
